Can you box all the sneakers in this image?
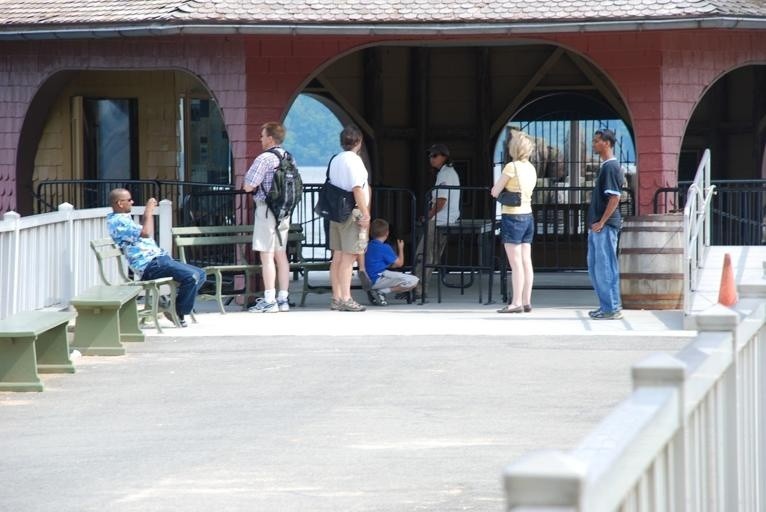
[589,307,603,317]
[159,295,172,321]
[276,297,290,312]
[370,289,388,306]
[591,310,624,320]
[330,297,339,310]
[367,290,379,305]
[249,298,280,313]
[339,297,367,311]
[173,316,187,328]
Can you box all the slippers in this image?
[358,271,372,291]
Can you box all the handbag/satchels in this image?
[314,154,366,223]
[498,162,521,207]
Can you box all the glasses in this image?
[120,198,132,202]
[428,153,442,158]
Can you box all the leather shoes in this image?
[405,294,428,300]
[395,291,413,300]
[497,305,523,313]
[523,304,531,312]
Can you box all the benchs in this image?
[0,310,76,392]
[69,282,147,357]
[89,237,198,335]
[170,222,331,315]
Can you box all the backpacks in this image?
[255,149,302,247]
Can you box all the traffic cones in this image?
[719,253,738,306]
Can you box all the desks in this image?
[435,218,503,304]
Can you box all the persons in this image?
[355,184,374,292]
[586,127,627,319]
[312,125,370,311]
[243,120,303,312]
[106,188,206,330]
[395,146,461,300]
[364,218,419,306]
[490,133,538,313]
